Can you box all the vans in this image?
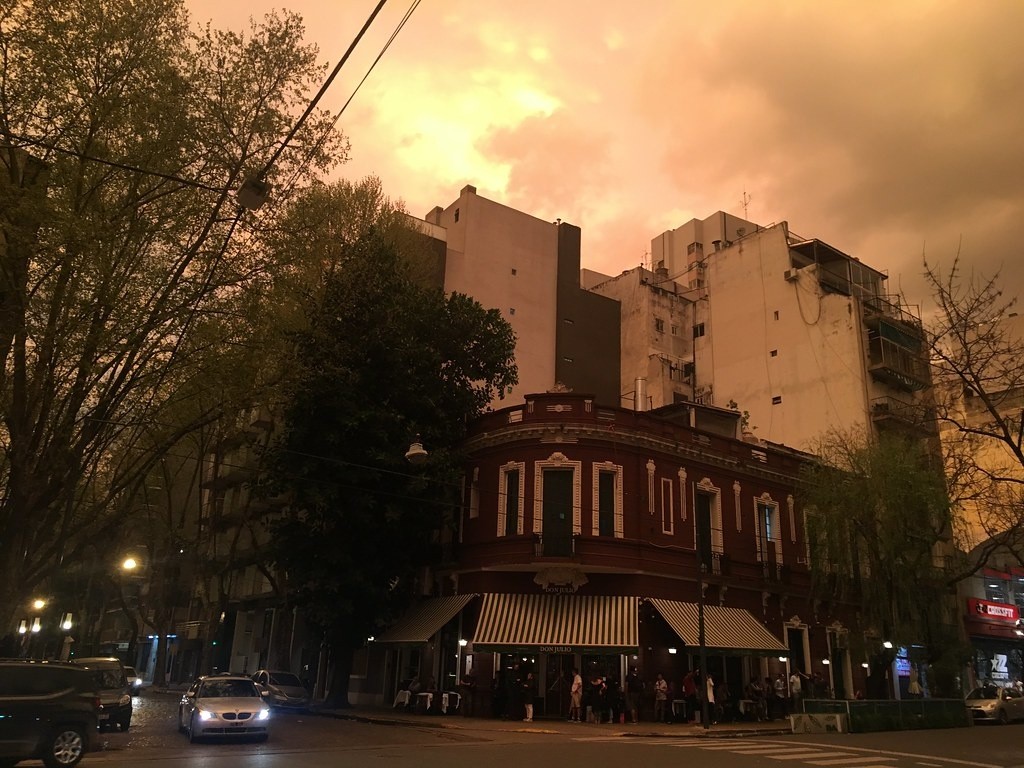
[72,657,134,734]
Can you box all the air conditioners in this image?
[783,267,798,281]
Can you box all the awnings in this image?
[647,597,792,651]
[471,592,640,648]
[372,593,480,646]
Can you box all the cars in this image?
[123,666,140,696]
[177,675,273,743]
[247,668,310,713]
[964,685,1024,726]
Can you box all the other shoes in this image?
[568,720,575,722]
[523,718,533,722]
[575,720,582,723]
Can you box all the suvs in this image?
[0,657,104,768]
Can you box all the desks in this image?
[392,690,460,715]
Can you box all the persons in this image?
[1013,678,1023,692]
[460,660,640,726]
[854,690,866,700]
[654,668,831,725]
[953,675,961,695]
[408,677,421,693]
